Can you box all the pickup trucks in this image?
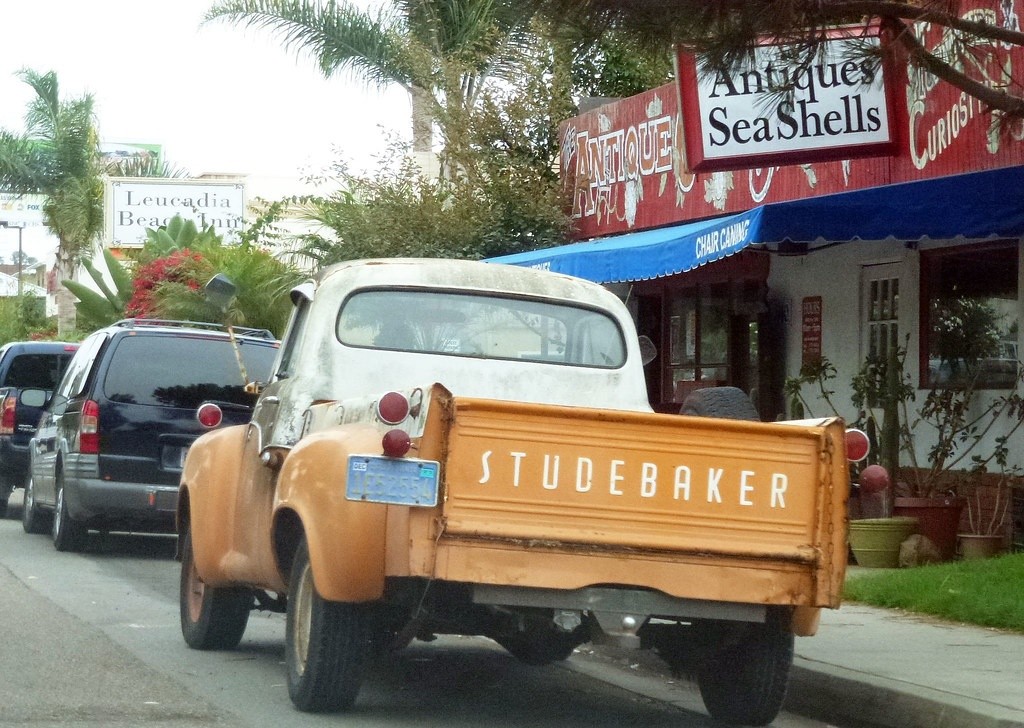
[175,255,852,727]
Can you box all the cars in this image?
[18,317,282,552]
[1,340,83,518]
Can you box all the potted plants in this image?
[781,333,1024,571]
